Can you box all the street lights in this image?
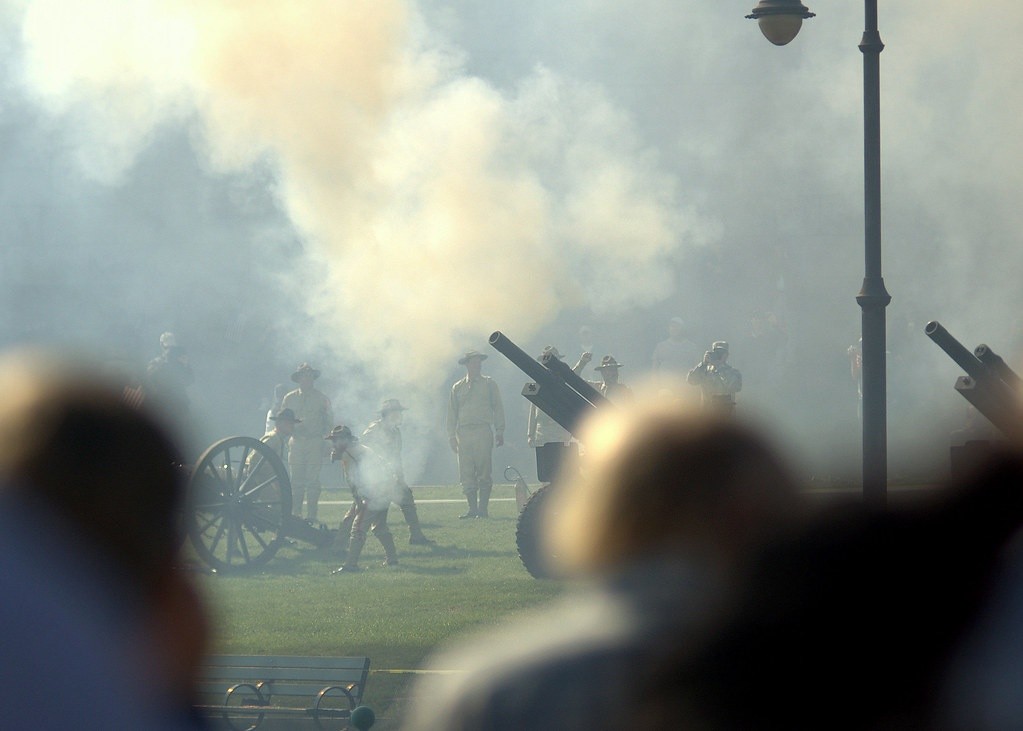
[747,1,891,508]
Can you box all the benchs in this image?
[188,652,375,731]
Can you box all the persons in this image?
[573,354,634,407]
[330,398,438,555]
[245,361,336,523]
[523,344,582,488]
[444,349,507,519]
[688,340,745,421]
[323,426,399,573]
[143,328,196,394]
[848,336,896,429]
[0,384,1023,730]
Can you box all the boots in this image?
[330,537,362,577]
[401,508,435,546]
[476,487,490,520]
[380,532,398,568]
[459,491,479,519]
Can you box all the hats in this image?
[269,408,301,422]
[458,350,488,364]
[291,361,320,383]
[595,355,624,371]
[160,331,175,347]
[376,399,409,414]
[325,426,358,441]
[537,345,565,363]
[713,341,729,351]
